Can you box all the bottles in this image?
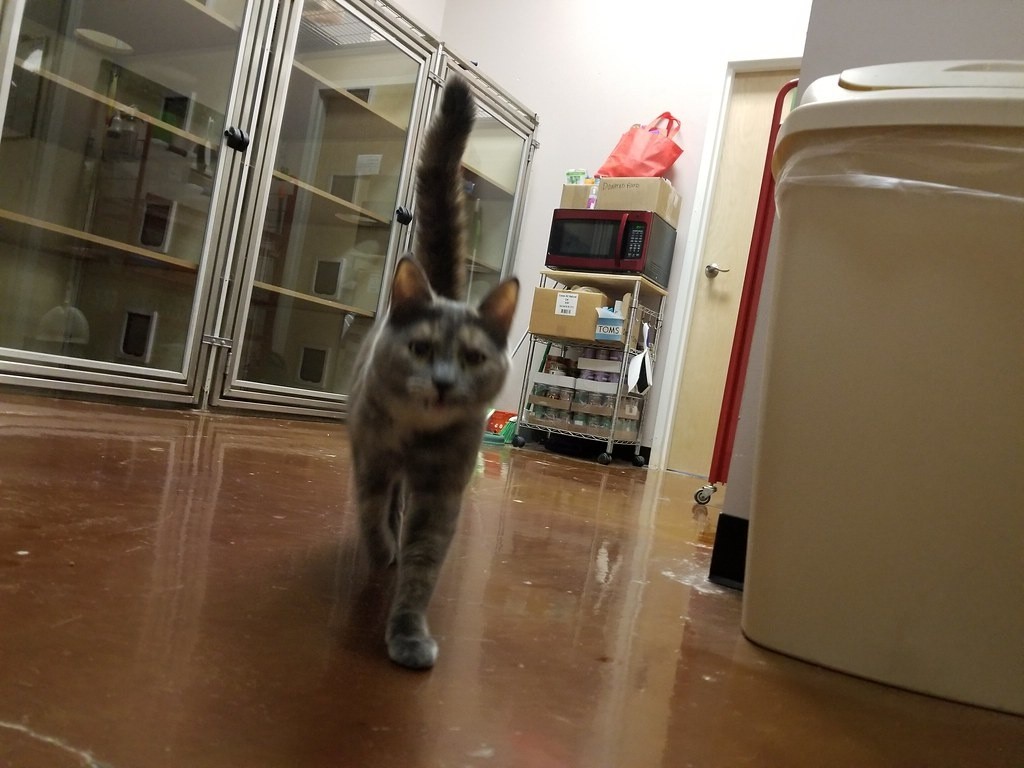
[586,178,601,209]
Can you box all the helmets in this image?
[35,305,90,346]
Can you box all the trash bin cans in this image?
[737,58,1024,714]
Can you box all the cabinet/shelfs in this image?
[0,0,541,418]
[513,271,670,468]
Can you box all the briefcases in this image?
[247,340,289,381]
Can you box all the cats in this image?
[344,74,520,668]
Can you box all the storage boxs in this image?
[560,184,599,209]
[567,424,637,440]
[577,357,630,375]
[571,402,639,421]
[533,371,576,388]
[528,395,571,410]
[575,378,628,396]
[595,177,682,229]
[527,287,612,342]
[528,415,569,429]
[595,293,643,345]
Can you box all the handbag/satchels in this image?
[627,322,653,399]
[592,111,684,180]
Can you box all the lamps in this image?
[35,265,90,344]
[72,15,138,56]
